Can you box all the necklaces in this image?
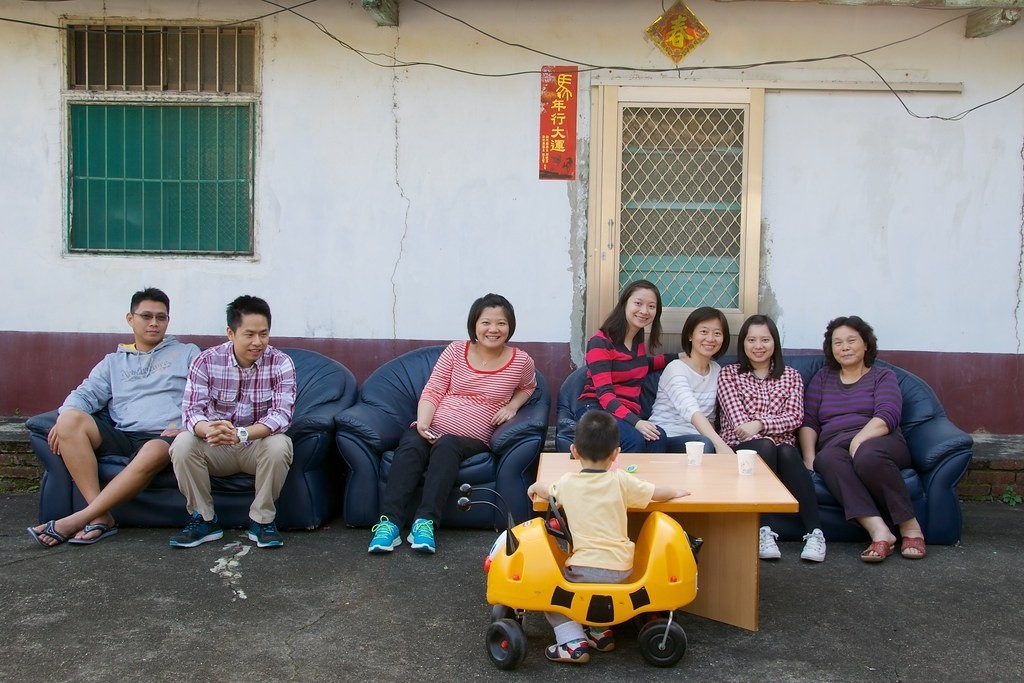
[475,341,498,367]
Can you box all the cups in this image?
[736,449,758,474]
[685,441,705,465]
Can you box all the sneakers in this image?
[406,520,437,553]
[249,521,283,549]
[544,640,590,663]
[585,628,616,652]
[169,511,223,548]
[368,515,403,552]
[758,527,782,559]
[801,530,827,562]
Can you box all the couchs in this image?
[334,344,549,532]
[558,353,974,545]
[25,345,357,528]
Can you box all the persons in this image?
[368,293,537,554]
[28,286,198,548]
[168,294,296,547]
[575,280,687,453]
[525,411,691,663]
[647,307,737,454]
[717,315,827,562]
[799,316,927,562]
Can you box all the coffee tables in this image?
[531,452,800,632]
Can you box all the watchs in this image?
[235,426,249,444]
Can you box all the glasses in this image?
[132,311,169,322]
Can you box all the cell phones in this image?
[425,430,439,439]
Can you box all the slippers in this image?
[67,522,120,544]
[901,535,926,559]
[27,520,66,548]
[861,540,895,562]
